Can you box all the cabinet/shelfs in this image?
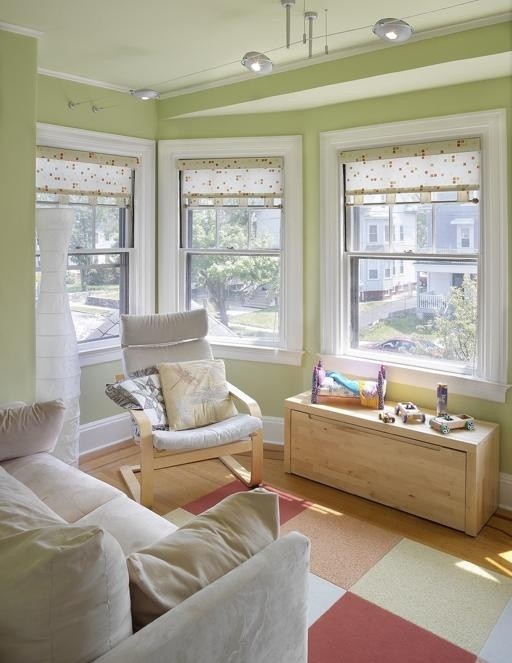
[280,386,502,538]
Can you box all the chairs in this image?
[113,307,266,508]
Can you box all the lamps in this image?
[133,88,159,102]
[240,50,273,76]
[281,0,329,58]
[371,16,414,44]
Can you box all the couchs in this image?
[0,400,311,661]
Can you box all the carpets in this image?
[157,478,512,662]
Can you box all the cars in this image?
[362,335,455,362]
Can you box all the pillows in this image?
[0,398,66,461]
[103,358,239,436]
